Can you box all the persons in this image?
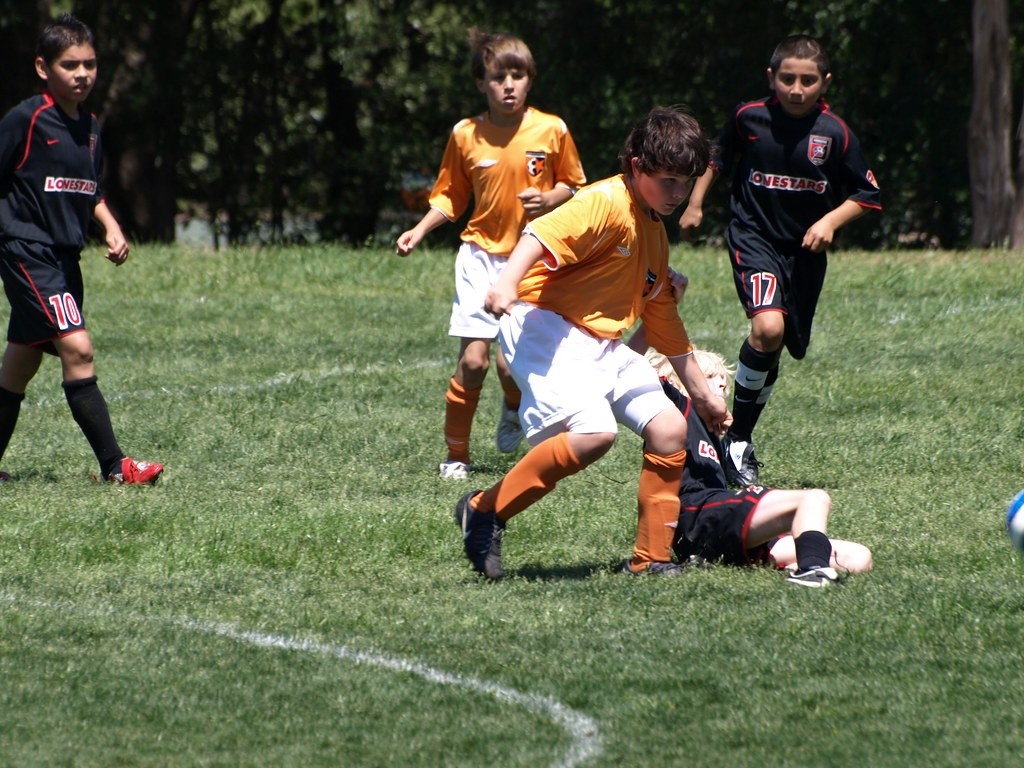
[456,107,733,579]
[679,36,881,484]
[627,268,872,589]
[396,38,587,479]
[0,13,163,484]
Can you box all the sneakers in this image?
[455,490,505,579]
[100,456,164,484]
[720,431,764,487]
[621,558,681,576]
[787,566,847,589]
[0,470,10,480]
[439,459,473,481]
[497,402,523,452]
